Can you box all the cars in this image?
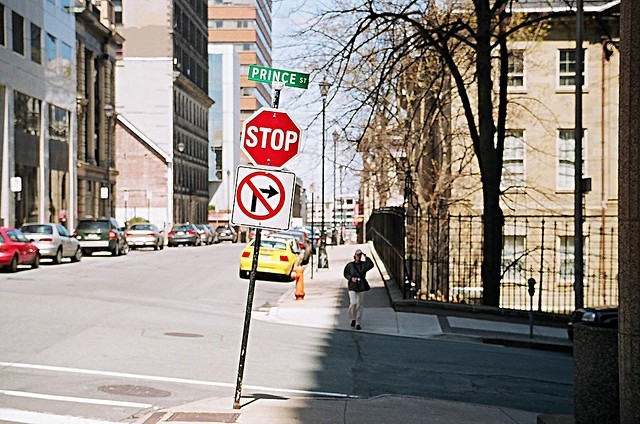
[126,223,164,250]
[196,224,213,244]
[296,225,322,248]
[19,222,82,263]
[568,306,617,340]
[277,230,309,264]
[320,223,343,247]
[168,223,201,246]
[239,237,300,280]
[0,225,40,272]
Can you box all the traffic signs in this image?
[248,64,310,88]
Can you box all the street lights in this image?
[339,164,345,244]
[332,129,340,245]
[318,73,333,267]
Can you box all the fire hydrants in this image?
[291,262,304,299]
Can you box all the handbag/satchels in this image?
[363,279,370,291]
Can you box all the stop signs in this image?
[240,106,302,170]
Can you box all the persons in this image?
[343,249,374,330]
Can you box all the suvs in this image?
[73,217,130,255]
[215,225,238,242]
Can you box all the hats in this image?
[356,249,363,254]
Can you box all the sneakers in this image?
[356,325,361,330]
[351,320,356,327]
